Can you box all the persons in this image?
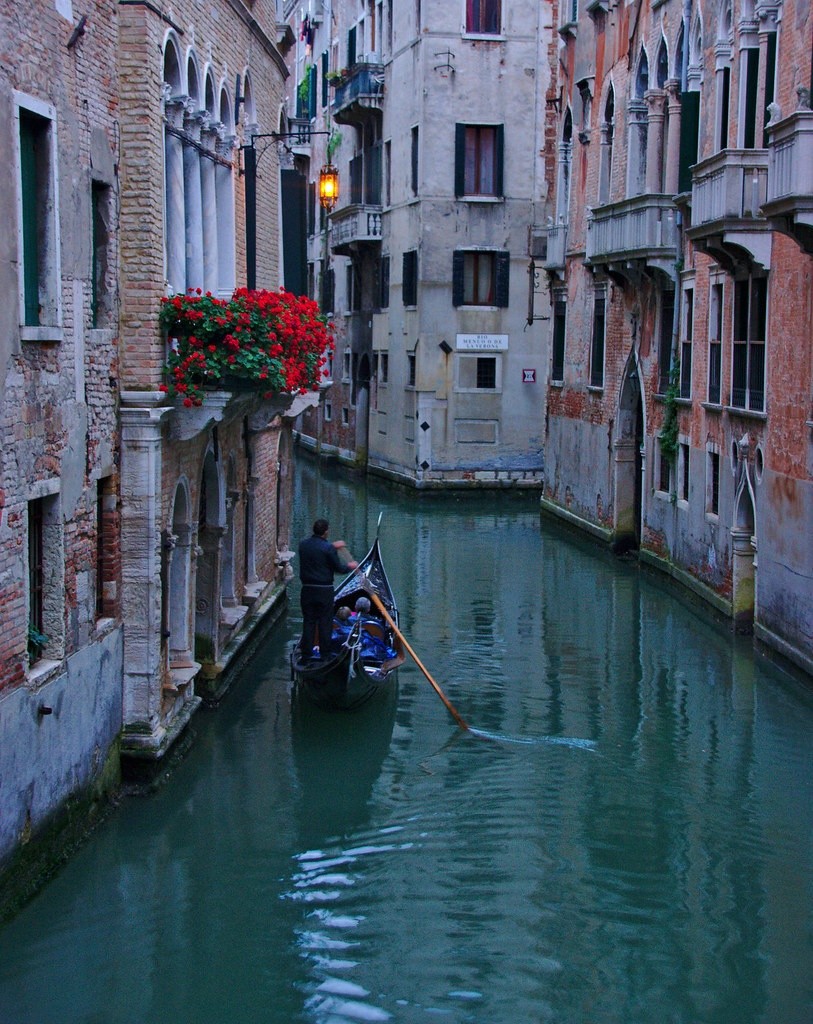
[332,597,392,648]
[297,519,358,666]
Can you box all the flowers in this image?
[158,284,337,409]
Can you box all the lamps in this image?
[250,132,338,214]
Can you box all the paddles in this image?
[342,546,468,732]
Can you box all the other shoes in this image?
[297,658,309,666]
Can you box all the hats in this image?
[355,597,371,609]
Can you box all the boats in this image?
[291,510,401,712]
